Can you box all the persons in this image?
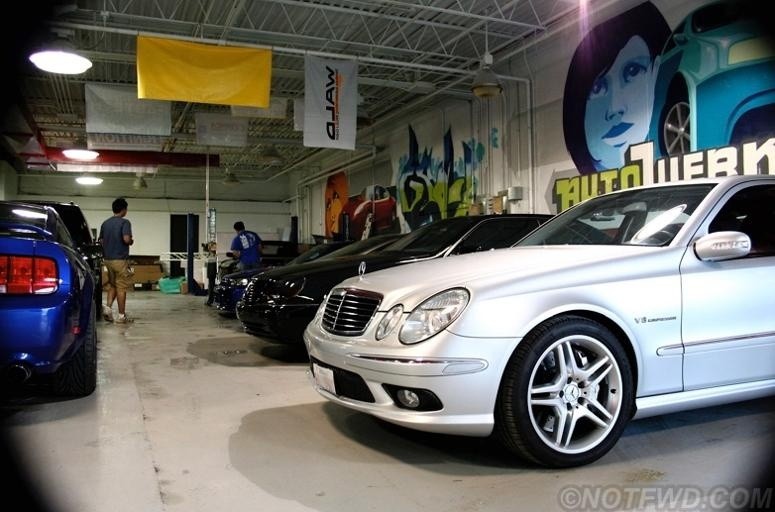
[230,223,265,270]
[100,197,134,323]
[564,1,674,176]
[326,171,346,236]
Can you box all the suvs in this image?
[4,200,106,322]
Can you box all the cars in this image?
[0,199,105,398]
[302,174,775,470]
[655,0,775,158]
[235,215,614,347]
[335,183,398,240]
[207,239,354,313]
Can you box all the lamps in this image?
[469,9,504,102]
[130,171,148,191]
[219,167,242,189]
[24,29,95,77]
[75,173,103,187]
[59,134,102,164]
[267,144,291,173]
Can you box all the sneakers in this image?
[116,314,134,323]
[104,307,113,321]
